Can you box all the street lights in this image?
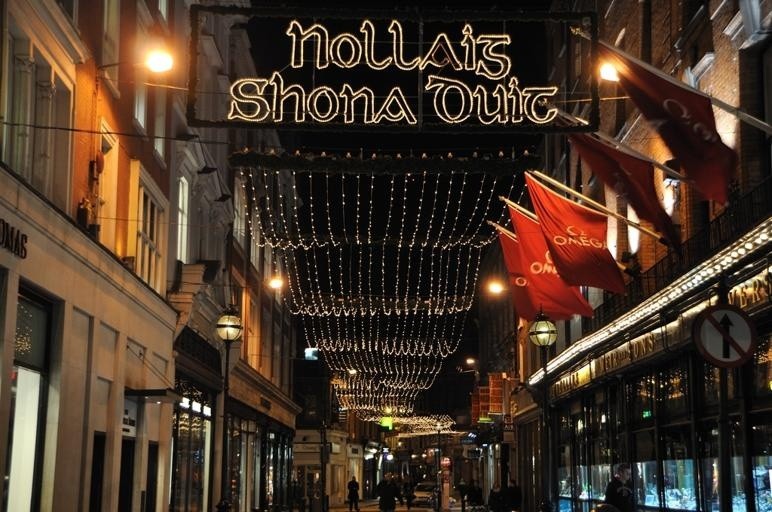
[435,419,444,471]
[216,304,243,512]
[528,311,558,512]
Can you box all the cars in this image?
[412,481,436,506]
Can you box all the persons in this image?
[505,478,523,512]
[604,460,635,511]
[467,479,483,506]
[489,480,506,511]
[346,476,360,511]
[376,471,417,511]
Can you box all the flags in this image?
[500,175,627,324]
[587,41,730,207]
[561,132,687,244]
